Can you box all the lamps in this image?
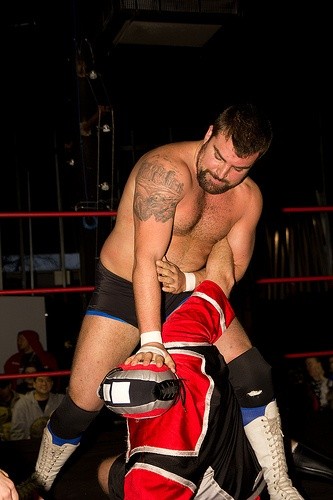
[80,103,111,138]
[73,36,100,80]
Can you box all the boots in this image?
[240,398,305,500]
[15,420,82,500]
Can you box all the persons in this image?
[14,103,304,500]
[288,357,333,457]
[0,329,124,441]
[98,236,267,500]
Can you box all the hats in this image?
[96,361,177,420]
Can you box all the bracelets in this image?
[183,273,195,292]
[141,331,162,346]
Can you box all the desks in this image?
[20,251,83,292]
